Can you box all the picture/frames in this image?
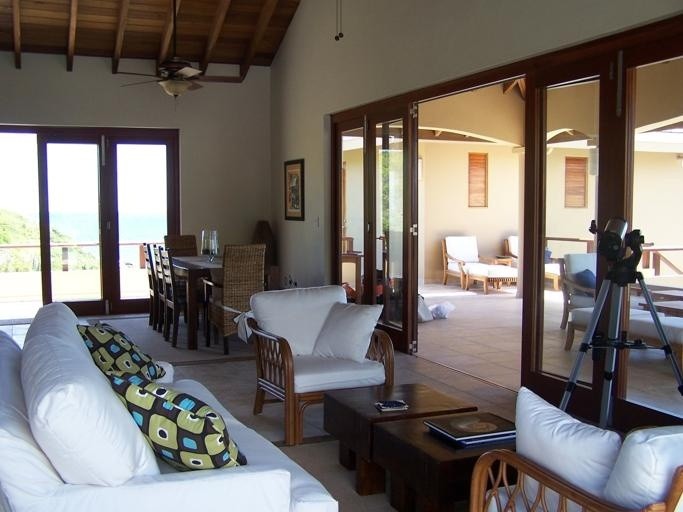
[282,158,305,221]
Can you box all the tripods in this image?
[560,263,683,430]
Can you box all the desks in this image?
[170,255,224,351]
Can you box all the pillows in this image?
[75,321,166,384]
[116,378,247,473]
[514,386,620,512]
[16,335,165,485]
[573,269,595,288]
[312,301,385,364]
[603,425,682,511]
[21,302,88,346]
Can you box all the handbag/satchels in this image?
[389,281,434,323]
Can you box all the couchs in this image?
[1,301,341,512]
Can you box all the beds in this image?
[441,239,518,295]
[638,300,682,316]
[562,305,683,368]
[640,268,683,290]
[504,237,569,290]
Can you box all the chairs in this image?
[143,242,188,331]
[160,250,219,347]
[232,284,395,447]
[556,257,598,330]
[163,234,214,336]
[204,244,266,356]
[153,244,199,338]
[468,424,682,511]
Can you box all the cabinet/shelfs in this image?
[342,252,363,291]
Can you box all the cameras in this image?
[592,215,650,265]
